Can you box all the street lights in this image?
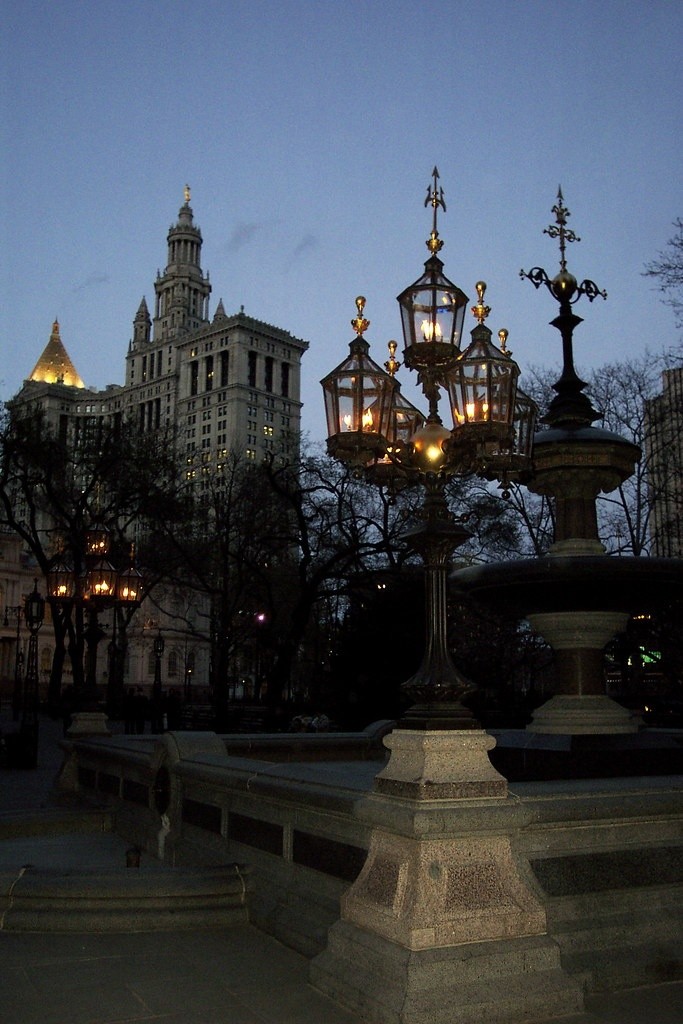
[46,483,145,800]
[308,163,582,1023]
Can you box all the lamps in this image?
[320,164,541,733]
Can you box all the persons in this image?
[276,710,329,733]
[122,687,148,734]
